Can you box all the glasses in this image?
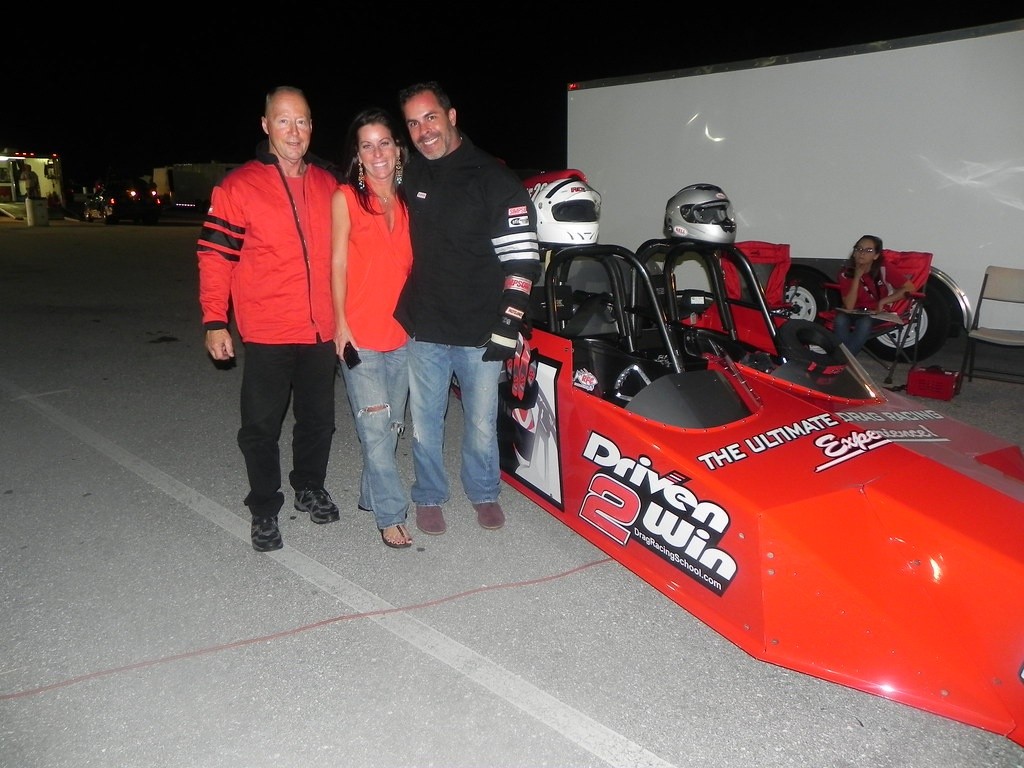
[852,246,877,253]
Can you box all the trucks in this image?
[0,153,69,213]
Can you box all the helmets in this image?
[527,175,602,245]
[664,183,736,243]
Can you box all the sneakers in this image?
[294,485,340,524]
[251,513,283,552]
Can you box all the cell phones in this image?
[343,340,361,370]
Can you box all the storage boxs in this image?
[906,366,959,400]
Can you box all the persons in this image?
[331,109,415,549]
[196,85,340,551]
[393,84,540,536]
[839,235,912,359]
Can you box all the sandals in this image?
[358,504,407,519]
[381,523,412,548]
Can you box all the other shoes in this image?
[473,501,505,530]
[415,504,447,535]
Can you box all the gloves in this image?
[476,304,531,362]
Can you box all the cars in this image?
[82,176,162,226]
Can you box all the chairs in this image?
[716,241,802,360]
[814,250,933,383]
[954,266,1024,397]
